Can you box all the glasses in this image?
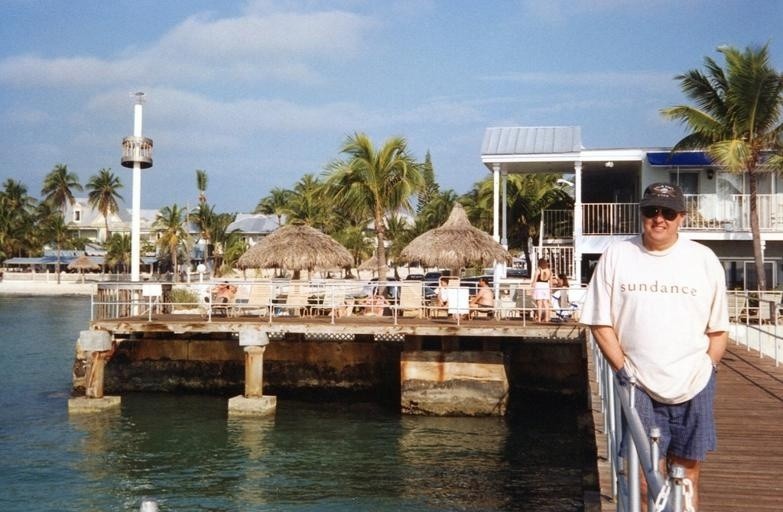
[640,206,678,221]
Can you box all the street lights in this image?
[124,85,157,315]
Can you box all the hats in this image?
[639,183,686,213]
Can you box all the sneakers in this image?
[222,298,228,306]
[204,297,209,303]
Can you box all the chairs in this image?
[681,200,733,228]
[770,202,783,228]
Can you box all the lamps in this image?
[604,161,614,168]
[707,169,713,179]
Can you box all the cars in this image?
[361,268,452,299]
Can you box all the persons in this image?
[436,278,448,309]
[531,257,553,323]
[209,281,234,308]
[458,278,494,320]
[551,272,569,306]
[574,180,729,511]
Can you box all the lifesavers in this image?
[551,288,578,316]
